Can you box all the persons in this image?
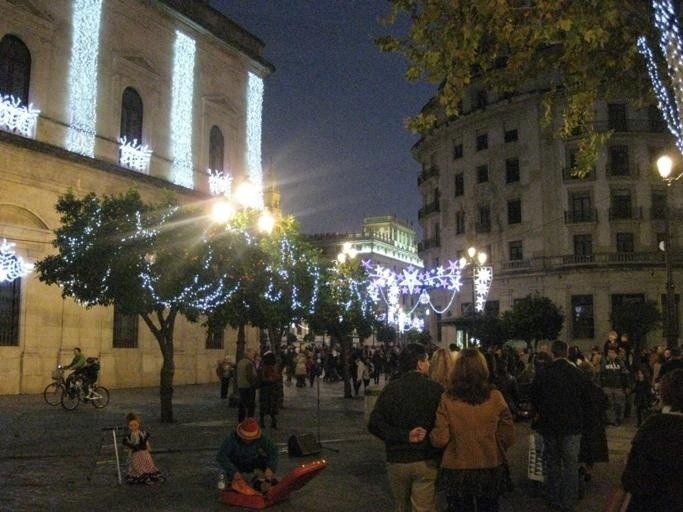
[122,413,166,486]
[620,368,682,509]
[218,417,278,490]
[64,348,85,387]
[280,341,401,396]
[367,337,610,510]
[603,332,682,427]
[70,358,97,399]
[219,344,283,430]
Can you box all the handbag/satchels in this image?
[527,431,546,483]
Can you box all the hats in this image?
[236,418,262,440]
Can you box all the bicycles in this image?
[61,380,110,411]
[44,363,73,406]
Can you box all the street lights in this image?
[460,246,488,310]
[337,242,357,270]
[656,154,683,350]
[210,185,274,405]
